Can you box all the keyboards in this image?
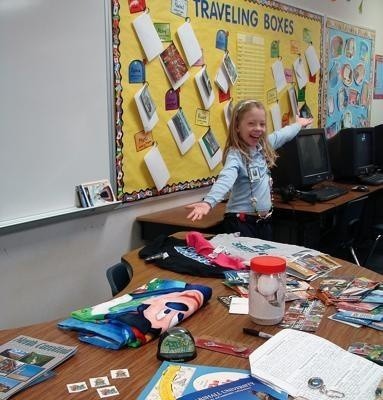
[303,186,348,201]
[362,172,383,185]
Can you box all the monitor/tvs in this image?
[270,128,333,192]
[326,128,375,179]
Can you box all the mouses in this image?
[352,185,369,192]
[377,168,383,173]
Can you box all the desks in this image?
[136,198,231,247]
[273,178,383,214]
[0,229,382,400]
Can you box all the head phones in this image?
[281,184,317,205]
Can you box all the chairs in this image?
[308,185,382,271]
[105,261,131,297]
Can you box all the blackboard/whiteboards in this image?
[0,1,376,239]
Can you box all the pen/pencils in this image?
[242,327,274,339]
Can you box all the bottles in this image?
[248,255,287,326]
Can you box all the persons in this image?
[185,98,313,241]
